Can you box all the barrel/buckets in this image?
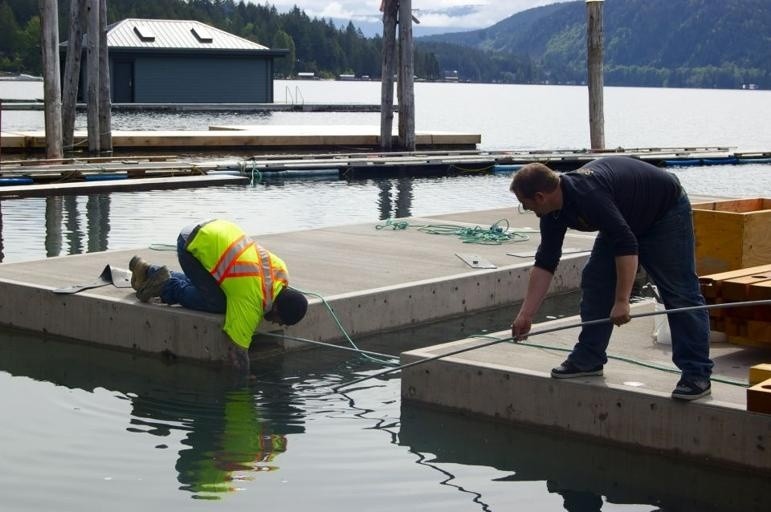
[651,297,672,345]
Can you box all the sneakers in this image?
[551,359,603,379]
[129,255,171,303]
[671,381,711,400]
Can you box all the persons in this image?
[129,218,308,376]
[509,154,714,402]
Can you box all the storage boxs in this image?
[691,199,771,276]
